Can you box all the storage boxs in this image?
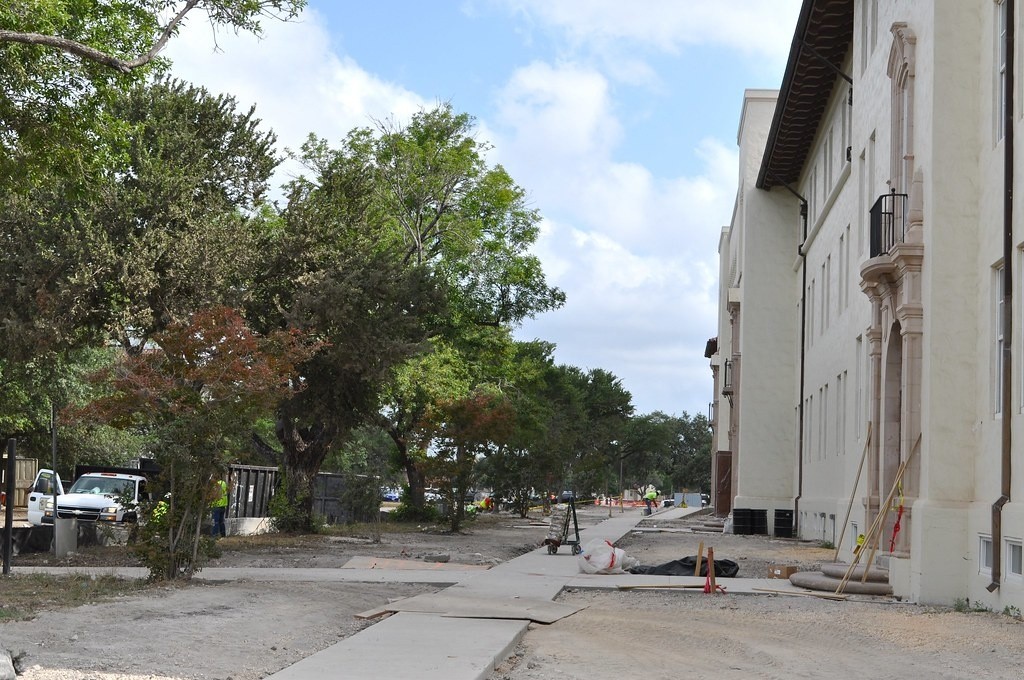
[768,564,797,580]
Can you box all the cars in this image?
[424,487,475,505]
[381,487,399,502]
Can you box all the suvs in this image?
[28,469,158,546]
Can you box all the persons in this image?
[643,490,661,514]
[207,474,228,537]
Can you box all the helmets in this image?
[657,490,661,495]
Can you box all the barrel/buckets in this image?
[644,509,648,516]
